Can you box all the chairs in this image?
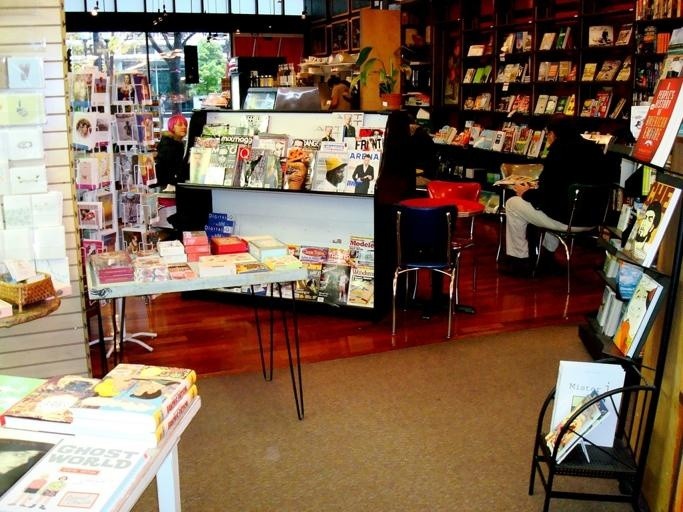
[536,182,611,293]
[426,179,481,304]
[391,198,461,340]
[495,163,542,267]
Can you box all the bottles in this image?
[253,63,296,87]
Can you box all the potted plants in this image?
[348,47,414,111]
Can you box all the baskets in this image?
[0,272,56,313]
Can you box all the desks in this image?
[84,240,308,420]
[0,369,180,512]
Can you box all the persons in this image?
[156,115,188,189]
[327,75,352,111]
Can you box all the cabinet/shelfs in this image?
[168,110,396,300]
[533,149,683,506]
[461,27,495,122]
[533,8,580,123]
[494,8,536,121]
[577,7,637,131]
[402,10,434,109]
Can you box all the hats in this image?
[326,157,347,173]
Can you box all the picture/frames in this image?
[304,0,370,56]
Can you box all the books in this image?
[545,360,626,464]
[0,361,197,512]
[68,72,157,255]
[432,2,683,358]
[189,112,386,307]
